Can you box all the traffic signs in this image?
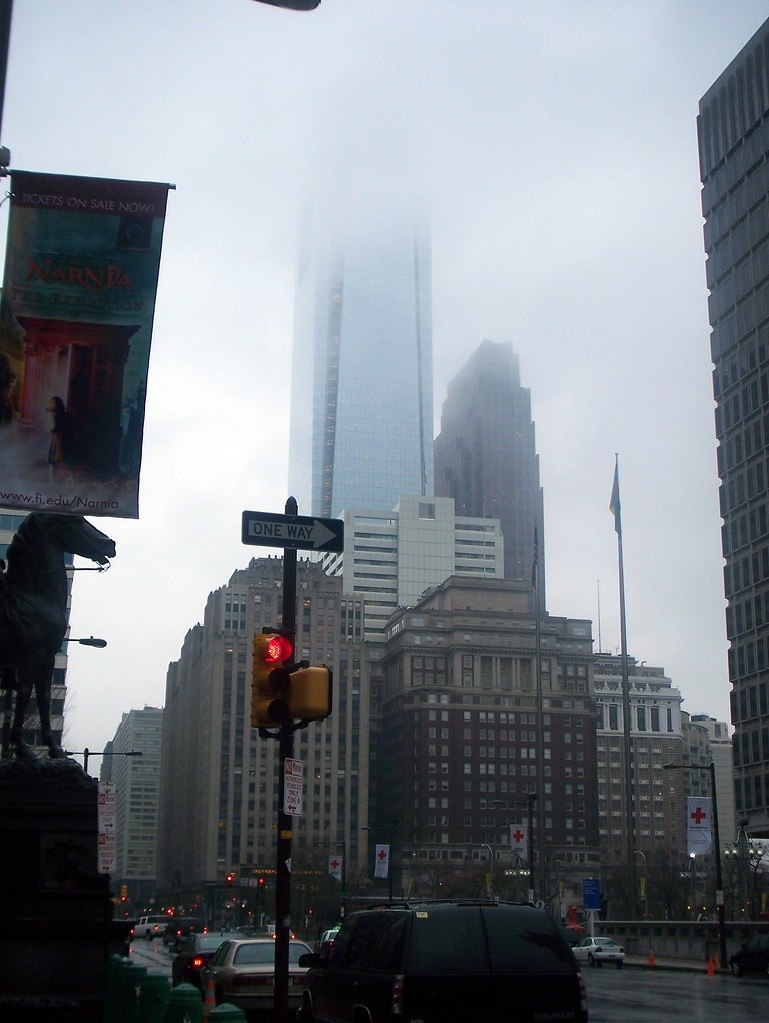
[241,510,346,554]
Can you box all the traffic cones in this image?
[648,951,656,967]
[707,955,715,976]
[203,979,216,1023]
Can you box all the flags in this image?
[610,460,622,539]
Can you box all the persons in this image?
[174,928,191,953]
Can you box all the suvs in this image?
[297,896,590,1023]
[164,916,205,952]
[132,914,174,942]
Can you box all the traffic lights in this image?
[228,876,232,881]
[121,885,127,902]
[250,632,296,728]
[259,877,264,885]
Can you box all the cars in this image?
[250,924,296,939]
[729,932,769,978]
[314,929,339,952]
[170,931,250,989]
[196,937,318,1023]
[571,936,626,968]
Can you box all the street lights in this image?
[64,747,143,775]
[662,759,728,970]
[633,850,648,922]
[315,840,346,924]
[690,852,696,920]
[2,635,107,758]
[748,849,764,922]
[491,792,534,903]
[481,843,493,899]
[724,849,738,922]
[361,826,393,903]
